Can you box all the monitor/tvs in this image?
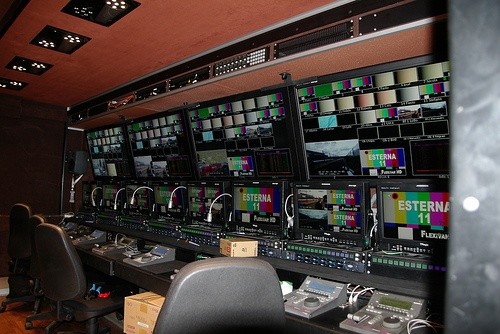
[81,51,453,257]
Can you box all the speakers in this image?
[72,150,89,175]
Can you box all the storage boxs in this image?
[124,292,165,334]
[220,238,258,257]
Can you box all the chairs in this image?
[152,257,286,334]
[0,203,135,334]
